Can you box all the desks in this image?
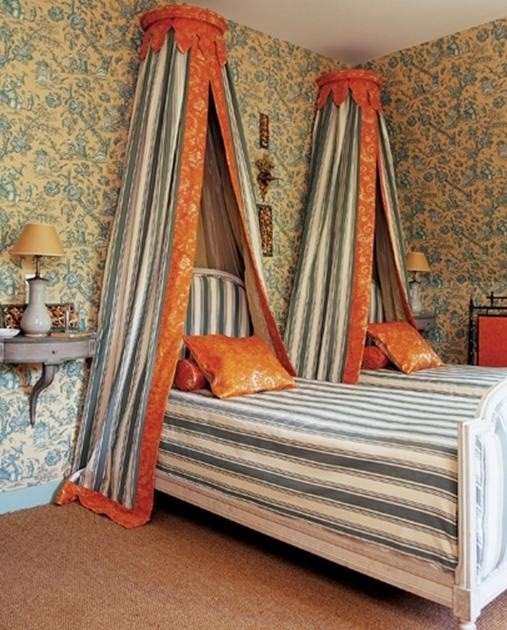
[0,330,95,425]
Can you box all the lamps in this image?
[406,251,431,317]
[11,221,64,337]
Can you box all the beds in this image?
[152,267,506,630]
[354,277,507,399]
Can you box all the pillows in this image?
[172,358,205,391]
[365,320,446,373]
[180,332,295,397]
[363,346,388,370]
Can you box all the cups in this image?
[65,310,79,333]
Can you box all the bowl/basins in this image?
[1,328,20,340]
